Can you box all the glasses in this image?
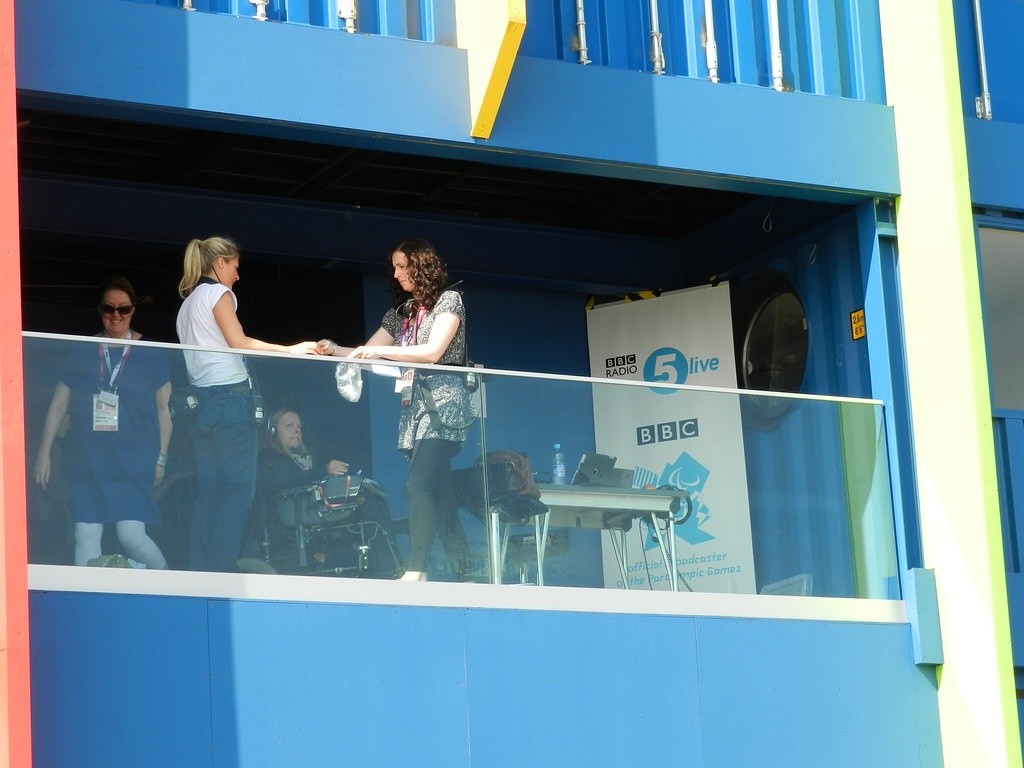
[99,304,134,315]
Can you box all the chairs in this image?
[257,454,409,578]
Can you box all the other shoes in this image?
[369,564,407,580]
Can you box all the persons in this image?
[33,279,173,570]
[176,237,325,573]
[316,238,475,583]
[261,404,403,580]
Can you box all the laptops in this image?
[571,454,617,485]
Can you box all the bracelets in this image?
[330,349,334,355]
[156,453,168,467]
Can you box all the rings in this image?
[365,351,370,355]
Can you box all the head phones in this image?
[655,484,692,525]
[267,412,277,436]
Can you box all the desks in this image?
[499,482,689,592]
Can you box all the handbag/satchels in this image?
[475,449,541,499]
[168,385,209,425]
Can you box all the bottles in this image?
[552,444,567,485]
